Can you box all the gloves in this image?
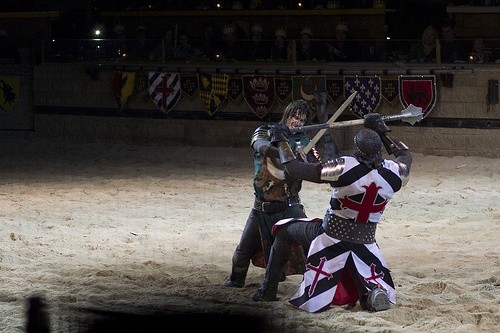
[363,111,391,136]
[268,123,291,143]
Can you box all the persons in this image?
[220,100,323,288]
[252,112,413,313]
[0,0,500,64]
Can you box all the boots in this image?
[250,246,292,301]
[363,288,391,310]
[223,258,250,287]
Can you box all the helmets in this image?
[353,128,384,165]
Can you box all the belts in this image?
[322,209,378,243]
[252,194,299,213]
